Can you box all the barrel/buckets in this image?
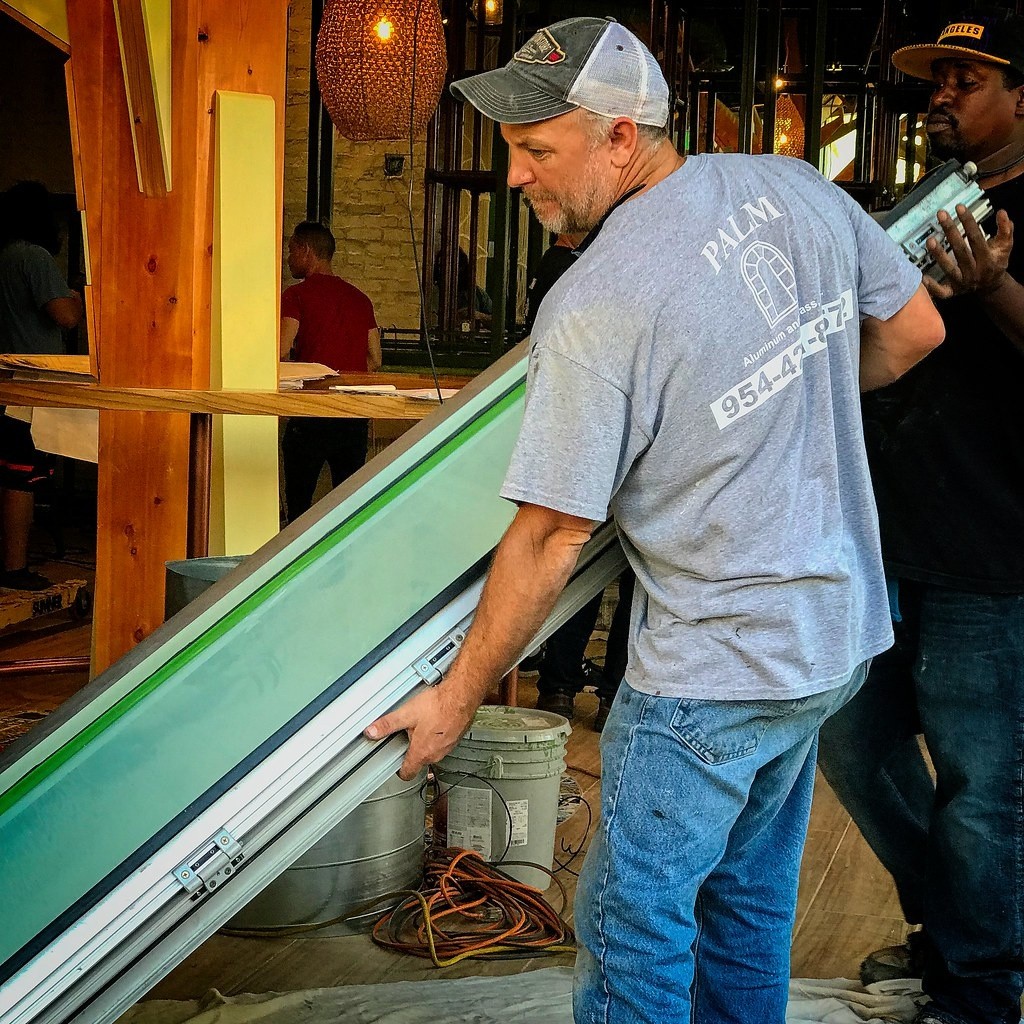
[435,706,572,895]
[165,555,432,942]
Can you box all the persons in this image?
[361,13,947,1024]
[528,568,637,735]
[280,220,383,523]
[430,245,493,330]
[1,179,86,590]
[520,231,602,694]
[814,0,1024,1024]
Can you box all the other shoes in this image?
[0,568,51,591]
[859,946,923,987]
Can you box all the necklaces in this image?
[978,154,1024,178]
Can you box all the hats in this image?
[450,17,670,129]
[890,9,1024,81]
